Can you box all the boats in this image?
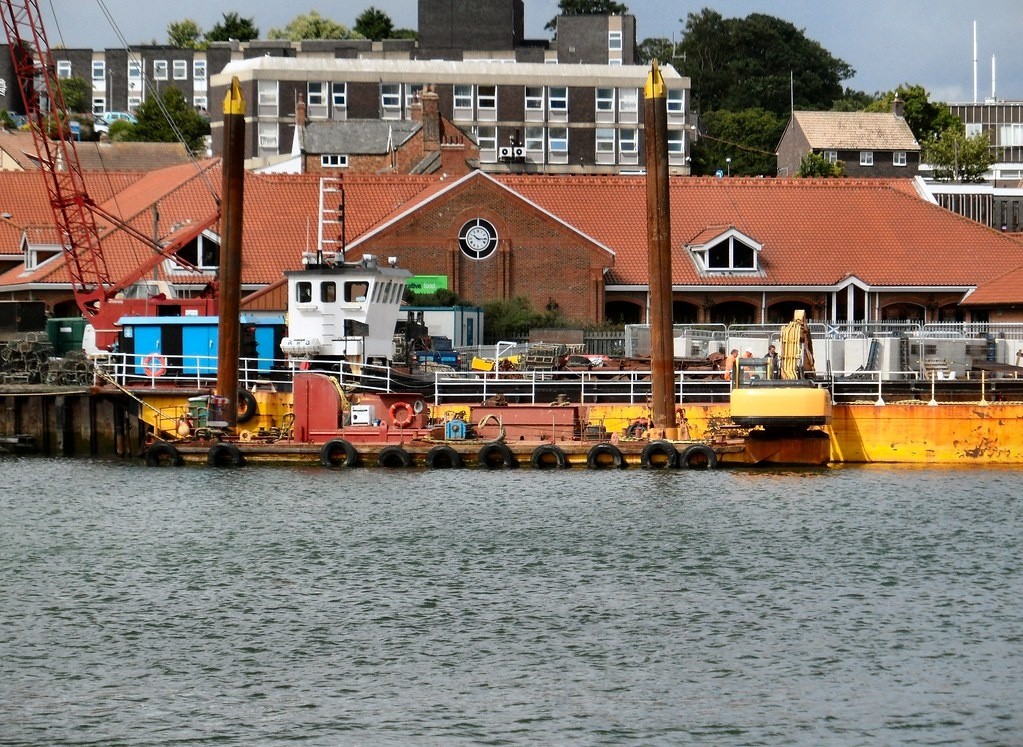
[84,53,833,470]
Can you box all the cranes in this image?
[1,0,290,385]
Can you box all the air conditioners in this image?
[498,147,512,157]
[513,147,526,158]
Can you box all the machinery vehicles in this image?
[728,308,833,431]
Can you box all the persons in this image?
[723,345,779,381]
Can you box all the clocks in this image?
[465,226,491,252]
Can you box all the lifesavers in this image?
[389,402,413,427]
[320,438,357,470]
[147,442,181,467]
[587,443,623,470]
[143,353,165,377]
[427,445,459,469]
[680,444,717,470]
[207,443,242,469]
[236,388,256,423]
[530,444,565,468]
[640,440,677,469]
[376,445,411,468]
[478,442,511,470]
